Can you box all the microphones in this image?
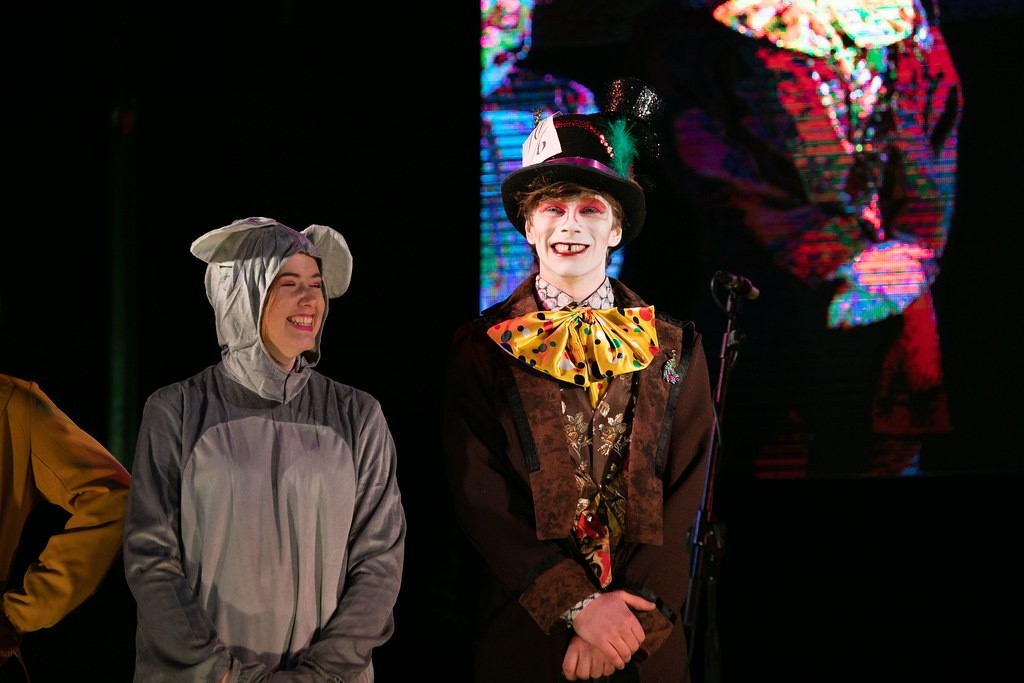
[717,270,759,299]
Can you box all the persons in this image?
[406,113,720,683]
[124,216,408,683]
[0,375,131,683]
[676,0,965,481]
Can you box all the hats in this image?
[499,112,646,253]
